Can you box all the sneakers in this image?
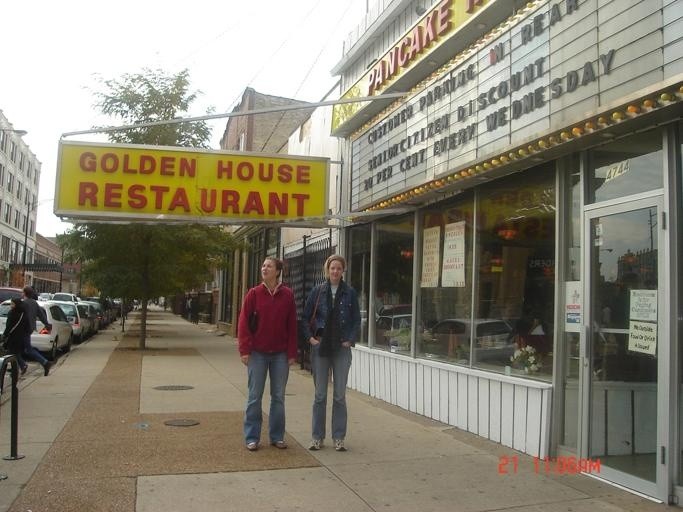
[309,439,324,450]
[333,439,347,451]
[246,442,258,450]
[20,362,52,377]
[274,440,288,449]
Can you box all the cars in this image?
[0,286,139,359]
[358,303,515,363]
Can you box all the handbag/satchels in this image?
[308,282,326,326]
[249,288,259,333]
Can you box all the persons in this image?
[186,295,200,324]
[300,254,361,449]
[19,284,54,377]
[235,256,297,450]
[2,296,28,376]
